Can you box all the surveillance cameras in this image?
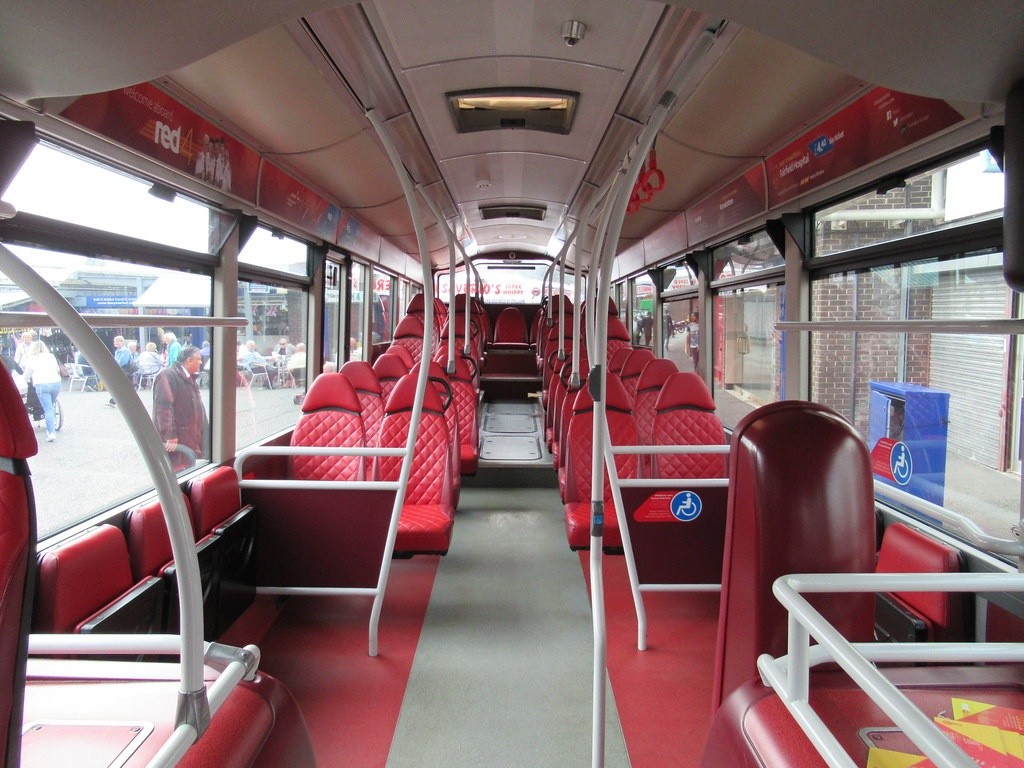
[560,21,586,47]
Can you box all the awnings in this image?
[0,291,34,311]
[134,274,214,308]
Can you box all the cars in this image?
[617,308,648,325]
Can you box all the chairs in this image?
[285,293,730,560]
[35,524,167,635]
[63,354,306,394]
[185,465,258,620]
[873,523,979,669]
[123,490,228,642]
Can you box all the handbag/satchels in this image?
[121,362,139,376]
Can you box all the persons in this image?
[199,335,306,388]
[632,309,674,351]
[153,346,210,474]
[128,340,139,358]
[24,341,61,442]
[14,331,51,373]
[136,342,166,377]
[108,335,137,407]
[161,331,182,368]
[685,310,699,372]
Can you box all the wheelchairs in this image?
[19,377,63,431]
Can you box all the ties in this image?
[189,375,193,385]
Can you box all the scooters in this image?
[672,315,689,334]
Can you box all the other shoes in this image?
[105,403,116,408]
[46,431,55,442]
